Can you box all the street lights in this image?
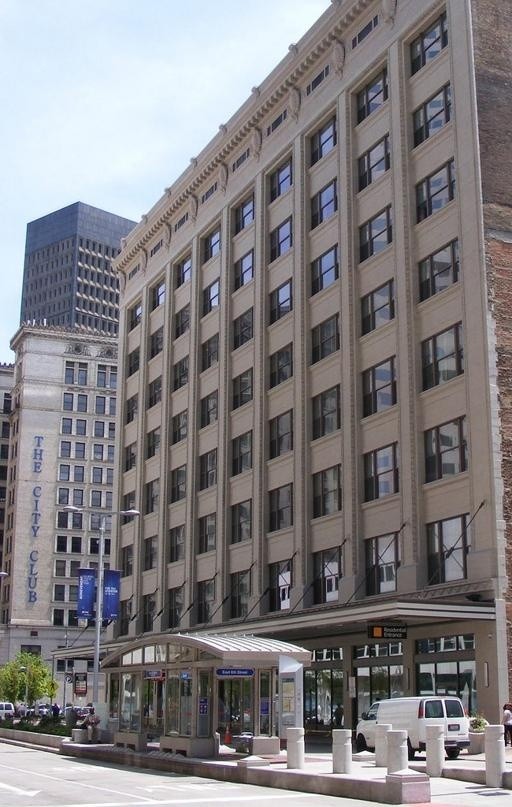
[63,506,140,702]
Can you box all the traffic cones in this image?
[223,726,233,744]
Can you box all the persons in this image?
[66,702,103,744]
[500,704,512,747]
[41,706,49,718]
[17,703,26,719]
[334,704,344,728]
[50,703,60,719]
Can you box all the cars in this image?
[0,703,93,720]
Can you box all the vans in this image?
[355,696,470,760]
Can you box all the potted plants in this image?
[467,705,489,755]
